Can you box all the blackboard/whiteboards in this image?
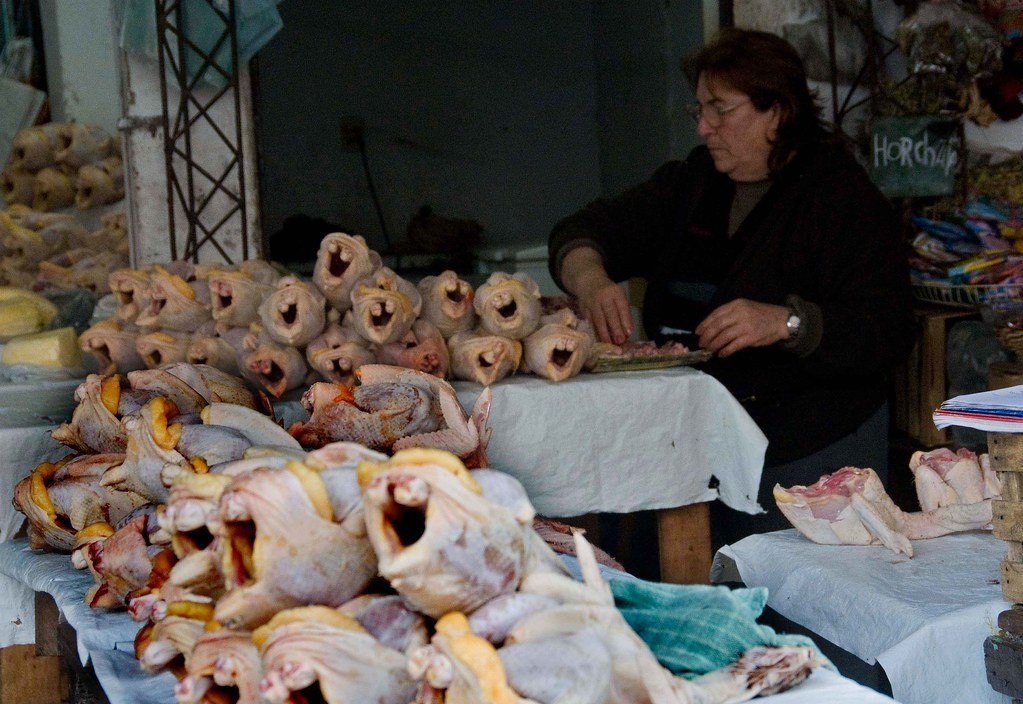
[871,115,963,196]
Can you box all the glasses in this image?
[683,96,750,121]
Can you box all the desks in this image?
[710,528,1016,704]
[0,363,771,704]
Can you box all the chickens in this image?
[0,121,1002,704]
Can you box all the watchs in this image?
[786,307,800,339]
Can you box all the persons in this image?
[543,28,925,515]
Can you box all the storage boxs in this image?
[894,303,1023,602]
